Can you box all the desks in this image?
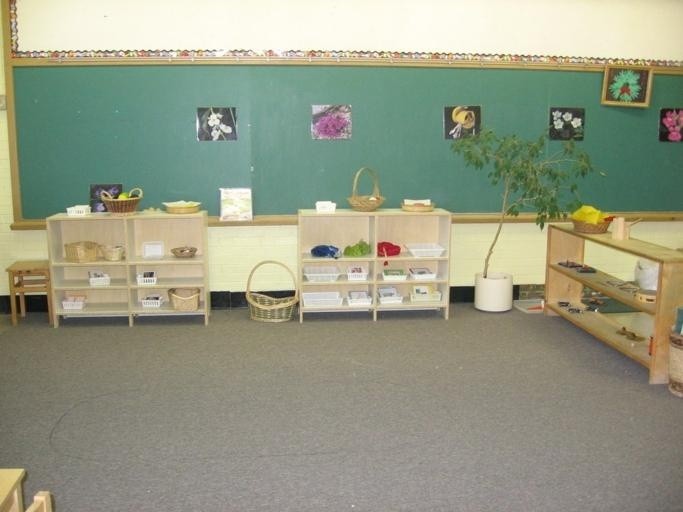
[0,468,26,512]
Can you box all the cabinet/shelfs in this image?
[46,211,211,331]
[544,222,683,386]
[299,206,453,323]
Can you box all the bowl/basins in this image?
[167,205,198,213]
[170,248,195,257]
[400,202,434,211]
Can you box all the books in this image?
[347,266,439,305]
[67,294,87,302]
[87,270,105,279]
[216,186,253,223]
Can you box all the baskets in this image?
[138,274,156,285]
[142,294,164,306]
[346,167,384,210]
[171,247,197,258]
[402,203,435,212]
[67,207,91,216]
[100,244,123,261]
[409,267,436,280]
[381,270,407,282]
[569,216,610,233]
[88,272,110,285]
[376,288,402,304]
[168,288,199,311]
[246,262,298,322]
[316,200,335,213]
[302,291,343,306]
[63,296,85,310]
[404,242,444,256]
[346,265,368,279]
[165,201,201,215]
[346,292,372,306]
[409,292,441,303]
[101,188,143,215]
[65,241,99,262]
[304,264,342,281]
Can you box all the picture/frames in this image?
[142,240,165,261]
[601,63,654,109]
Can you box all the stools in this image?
[5,260,53,326]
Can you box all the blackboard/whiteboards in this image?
[3,51,683,231]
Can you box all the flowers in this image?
[205,108,232,140]
[449,108,475,137]
[312,111,349,140]
[663,110,683,141]
[552,110,583,137]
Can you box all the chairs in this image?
[24,491,53,512]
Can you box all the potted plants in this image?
[449,128,607,314]
